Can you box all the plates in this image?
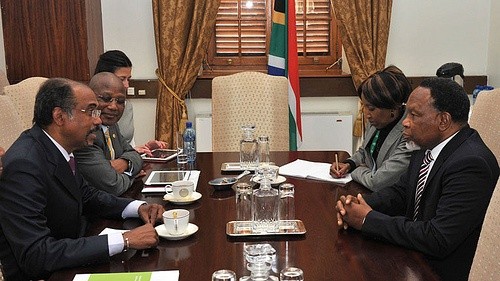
[252,174,286,186]
[163,192,202,204]
[154,223,199,241]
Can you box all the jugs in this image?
[239,124,259,169]
[252,166,279,234]
[239,244,280,281]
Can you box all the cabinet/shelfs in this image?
[0,0,104,86]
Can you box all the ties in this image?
[69,157,76,176]
[105,128,115,161]
[414,151,433,221]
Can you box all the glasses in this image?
[94,92,128,107]
[54,106,102,120]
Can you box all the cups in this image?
[255,165,279,181]
[279,183,295,233]
[258,134,270,170]
[211,269,237,281]
[235,183,253,234]
[165,181,194,200]
[176,131,189,164]
[162,209,191,235]
[280,266,304,281]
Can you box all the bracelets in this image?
[362,218,366,224]
[123,232,129,248]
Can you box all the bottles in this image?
[184,122,196,161]
[472,84,494,104]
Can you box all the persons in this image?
[335,76,500,281]
[330,65,422,192]
[0,78,166,281]
[73,72,145,197]
[94,50,168,156]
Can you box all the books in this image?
[278,158,353,184]
[72,270,179,281]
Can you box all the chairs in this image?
[212,71,289,152]
[468,89,500,281]
[0,77,49,153]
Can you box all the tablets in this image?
[148,169,191,185]
[140,148,184,160]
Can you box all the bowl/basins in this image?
[207,177,239,189]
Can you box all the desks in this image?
[45,150,446,281]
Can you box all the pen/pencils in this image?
[335,153,339,174]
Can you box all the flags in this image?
[268,0,302,151]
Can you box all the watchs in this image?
[129,161,131,172]
